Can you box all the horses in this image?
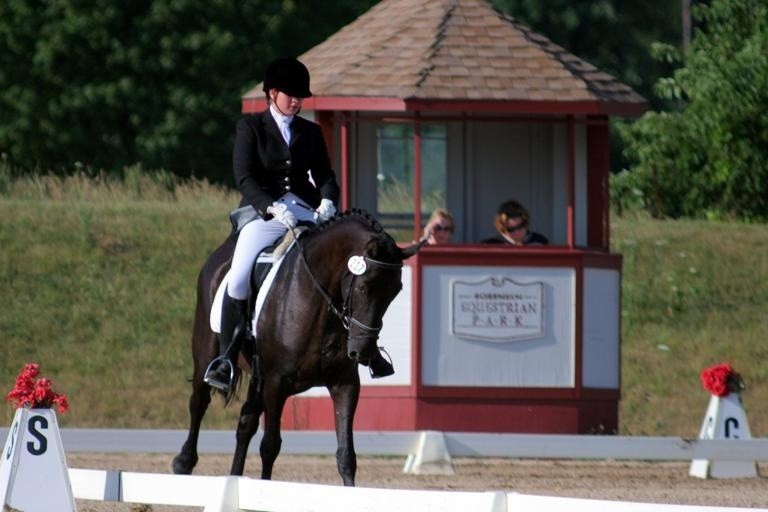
[169,205,429,487]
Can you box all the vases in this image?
[690,393,760,480]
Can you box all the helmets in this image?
[262,57,312,99]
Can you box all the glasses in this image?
[432,224,451,232]
[507,221,525,232]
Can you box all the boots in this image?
[358,336,395,376]
[204,282,249,384]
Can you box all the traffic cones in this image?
[684,377,759,479]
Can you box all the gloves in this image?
[266,201,298,228]
[313,198,336,222]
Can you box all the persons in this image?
[422,209,455,246]
[479,198,550,246]
[205,53,394,390]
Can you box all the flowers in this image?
[699,363,746,397]
[6,363,70,413]
